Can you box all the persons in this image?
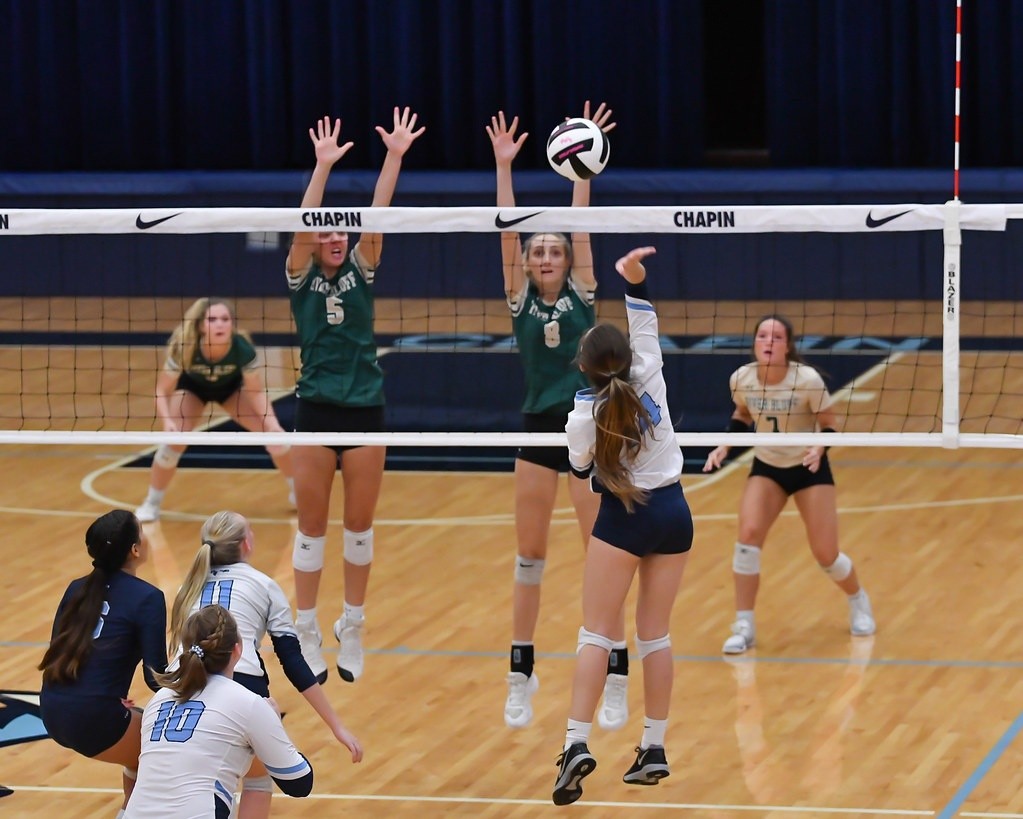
[122,605,313,819]
[286,106,426,685]
[132,296,298,523]
[486,101,632,728]
[703,314,874,654]
[39,511,170,819]
[553,246,694,806]
[168,510,364,819]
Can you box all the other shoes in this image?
[289,491,299,504]
[136,495,161,520]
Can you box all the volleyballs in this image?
[546,118,610,181]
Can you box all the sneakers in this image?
[296,618,328,685]
[623,742,671,785]
[722,618,754,654]
[851,591,874,635]
[334,612,365,682]
[552,741,597,807]
[504,672,539,726]
[598,673,628,728]
